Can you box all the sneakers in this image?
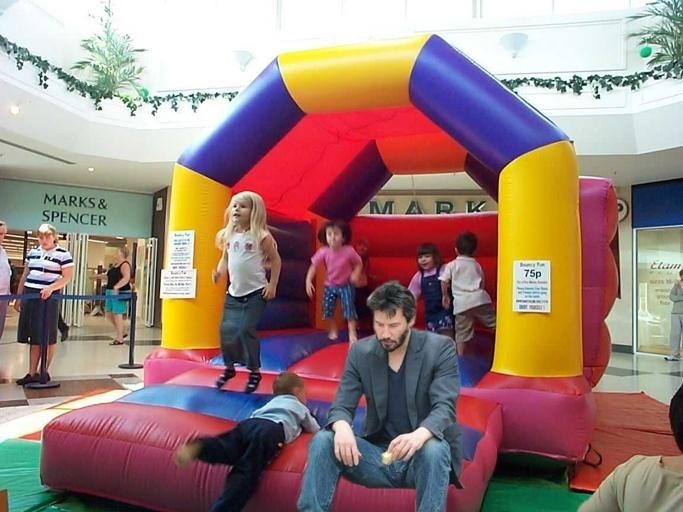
[664,356,680,361]
[16,372,50,385]
[61,326,69,341]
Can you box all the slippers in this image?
[109,334,128,345]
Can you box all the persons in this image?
[100,243,135,345]
[578,382,683,512]
[13,224,74,385]
[174,373,320,512]
[305,221,364,346]
[665,269,683,361]
[438,231,496,356]
[8,260,15,302]
[295,281,461,512]
[51,240,70,341]
[212,190,282,390]
[406,242,456,342]
[0,221,14,383]
